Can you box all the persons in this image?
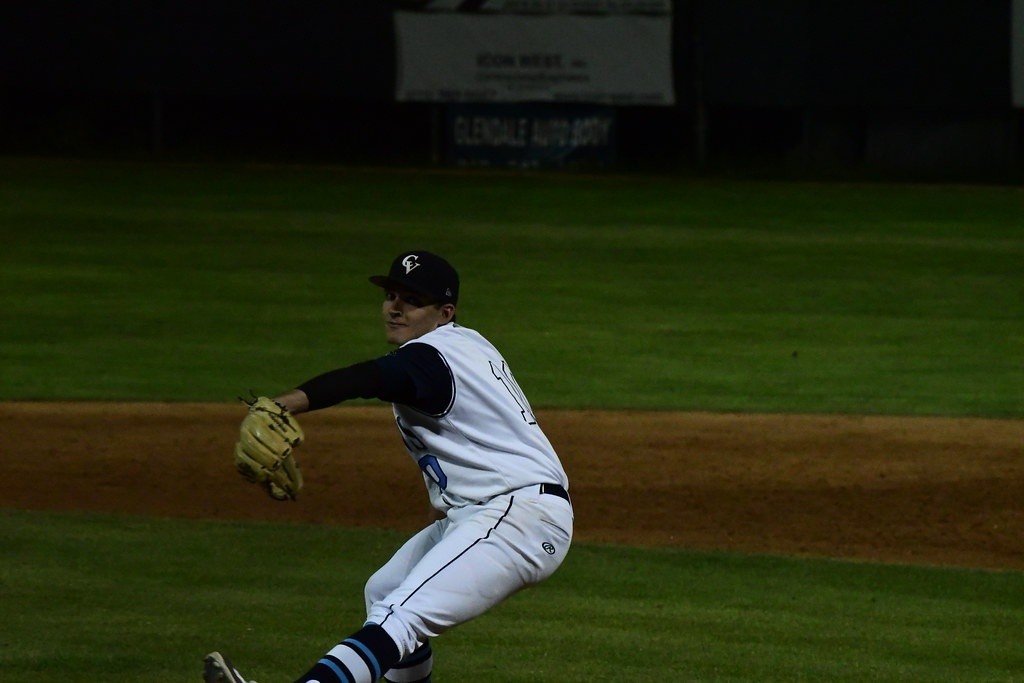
[203,251,573,683]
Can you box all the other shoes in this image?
[203,651,250,683]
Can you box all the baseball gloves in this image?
[233,395,307,500]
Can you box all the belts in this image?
[539,484,571,504]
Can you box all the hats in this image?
[369,250,458,305]
